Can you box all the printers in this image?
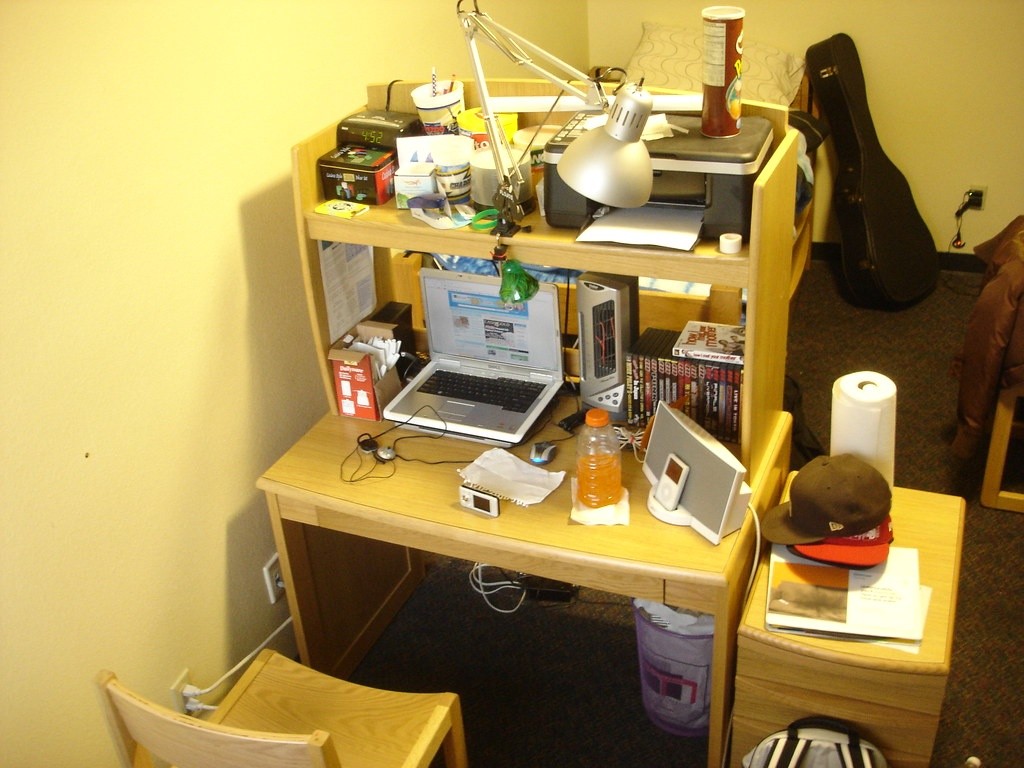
[542,114,774,242]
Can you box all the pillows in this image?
[623,20,806,109]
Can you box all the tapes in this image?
[720,233,742,254]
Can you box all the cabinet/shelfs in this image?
[258,77,794,768]
[730,471,968,768]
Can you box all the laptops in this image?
[382,266,567,445]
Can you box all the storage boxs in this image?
[394,162,439,209]
[327,320,402,423]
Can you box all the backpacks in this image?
[741,716,889,768]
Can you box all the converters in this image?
[521,576,573,603]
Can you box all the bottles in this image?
[576,408,622,508]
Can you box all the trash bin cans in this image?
[631,597,715,738]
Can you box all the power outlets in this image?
[968,185,988,210]
[170,667,199,718]
[263,552,285,604]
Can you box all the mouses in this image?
[529,441,557,465]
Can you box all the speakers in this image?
[641,399,750,546]
[577,269,639,422]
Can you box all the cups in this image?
[411,80,465,135]
[456,107,518,150]
[435,158,472,210]
[513,125,563,198]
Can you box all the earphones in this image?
[357,434,396,464]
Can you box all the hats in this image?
[760,453,892,545]
[794,514,893,566]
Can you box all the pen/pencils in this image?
[432,66,437,96]
[450,74,455,91]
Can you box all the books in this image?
[625,320,745,447]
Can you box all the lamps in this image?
[455,0,655,240]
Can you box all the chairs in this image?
[96,649,469,768]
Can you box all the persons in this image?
[730,336,745,356]
[719,340,745,354]
[682,349,692,359]
